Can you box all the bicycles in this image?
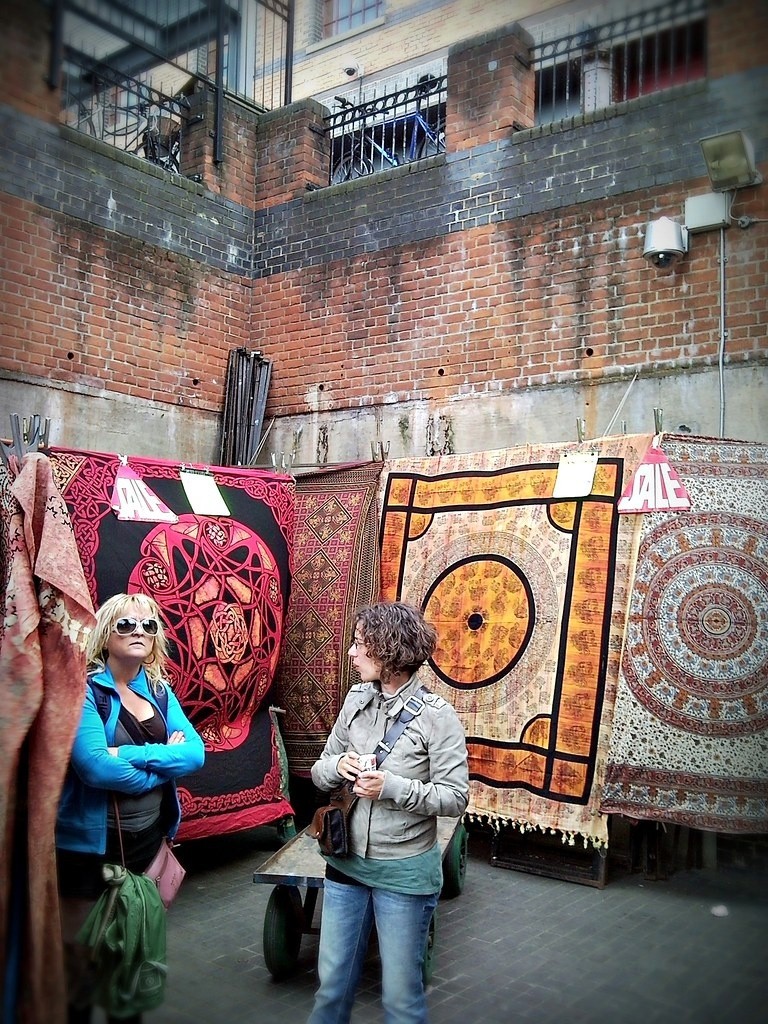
[132,113,204,184]
[321,74,445,187]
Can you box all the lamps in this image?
[699,129,762,192]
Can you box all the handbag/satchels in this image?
[142,838,186,910]
[305,805,348,858]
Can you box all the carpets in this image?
[0,433,768,1024]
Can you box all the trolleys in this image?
[248,802,470,998]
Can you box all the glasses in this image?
[106,617,159,637]
[351,639,366,650]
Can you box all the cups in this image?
[359,754,376,781]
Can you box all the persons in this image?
[306,603,471,1024]
[54,596,204,1024]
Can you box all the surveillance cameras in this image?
[642,215,684,270]
